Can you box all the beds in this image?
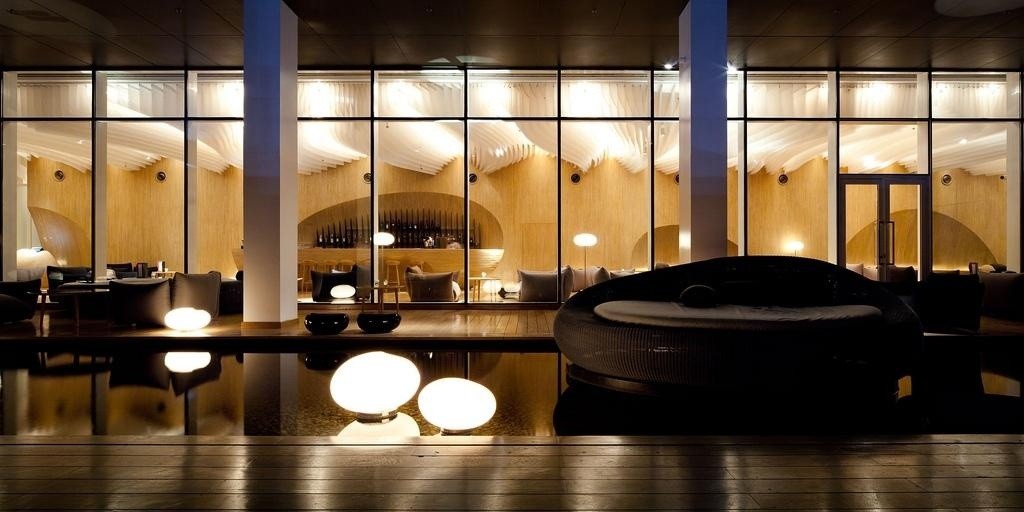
[549,256,924,413]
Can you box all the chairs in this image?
[876,263,1023,322]
[0,257,243,329]
